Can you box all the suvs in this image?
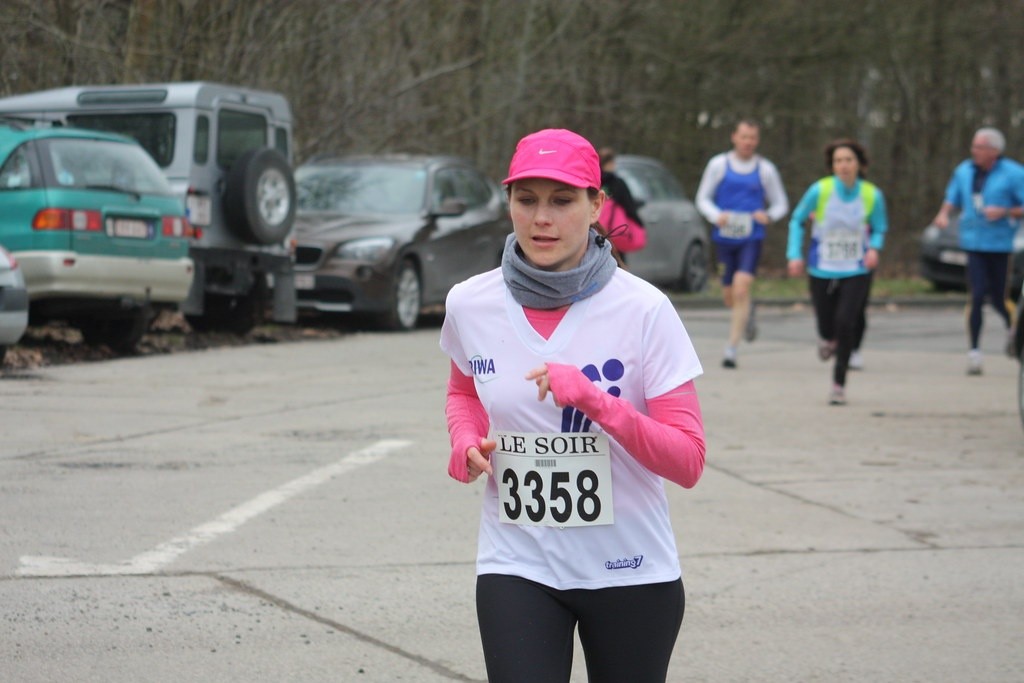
[0,81,299,334]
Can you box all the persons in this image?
[930,127,1023,374]
[1007,230,1024,432]
[598,148,647,270]
[440,128,707,683]
[695,115,789,369]
[786,137,889,406]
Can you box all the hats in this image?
[501,128,600,191]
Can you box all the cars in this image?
[279,151,514,335]
[604,155,713,297]
[1,121,197,351]
[915,209,966,293]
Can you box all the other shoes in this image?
[723,349,736,366]
[745,320,758,338]
[831,390,846,404]
[820,346,833,359]
[966,352,982,373]
